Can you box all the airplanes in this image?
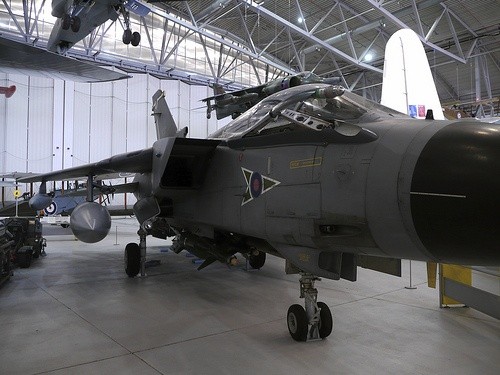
[46,0,140,53]
[0,72,500,345]
